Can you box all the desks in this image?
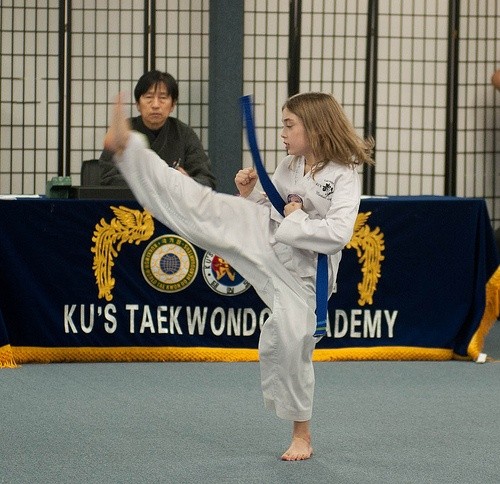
[0,194,500,367]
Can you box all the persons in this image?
[100,71,215,192]
[103,92,375,460]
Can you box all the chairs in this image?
[81,160,99,187]
